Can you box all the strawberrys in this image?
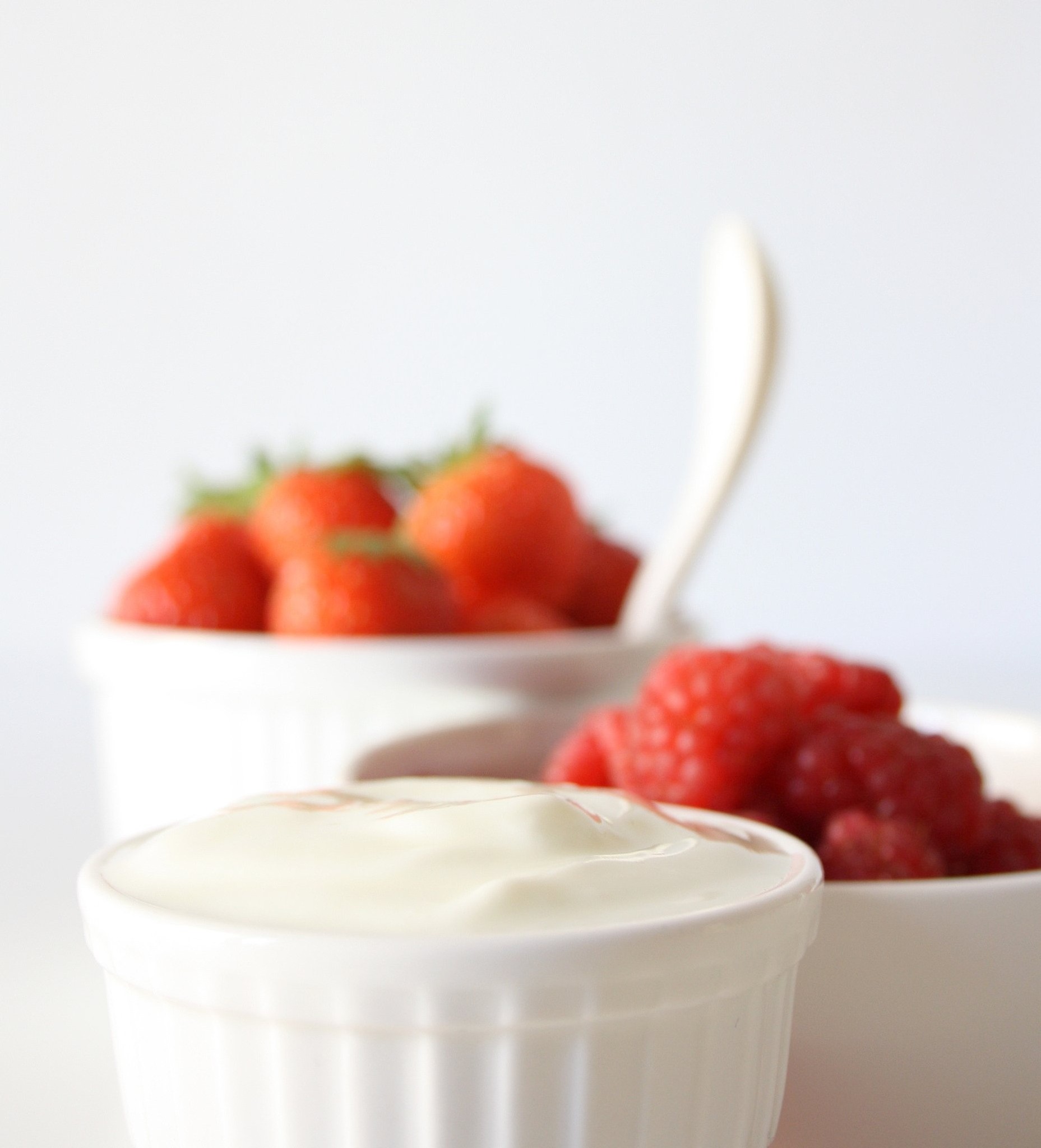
[106,415,639,640]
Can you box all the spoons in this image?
[627,213,783,649]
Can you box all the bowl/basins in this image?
[65,597,702,838]
[88,780,820,1148]
[357,720,1040,1148]
